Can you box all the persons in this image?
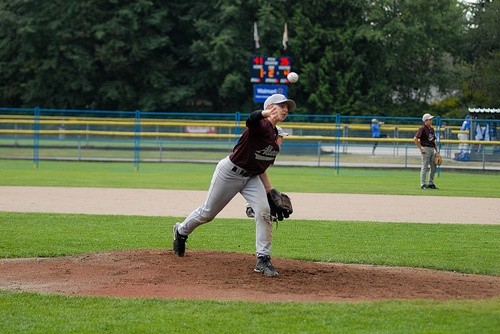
[173,93,296,277]
[458,114,471,153]
[245,126,289,217]
[413,114,439,188]
[371,119,390,156]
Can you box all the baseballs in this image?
[287,72,299,83]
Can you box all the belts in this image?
[232,166,249,177]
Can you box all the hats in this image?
[264,94,296,112]
[423,114,435,122]
[466,115,470,119]
[372,119,378,122]
[276,126,289,136]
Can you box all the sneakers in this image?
[172,225,188,257]
[254,255,279,277]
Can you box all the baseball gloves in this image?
[267,188,293,222]
[433,152,442,168]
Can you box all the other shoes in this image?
[273,218,276,221]
[421,184,436,190]
[246,207,255,217]
